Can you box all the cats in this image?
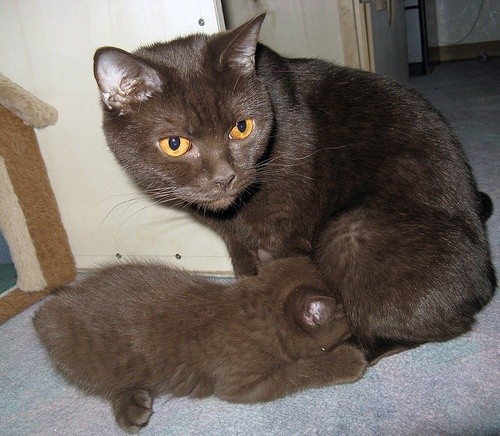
[31,246,368,435]
[92,11,498,366]
